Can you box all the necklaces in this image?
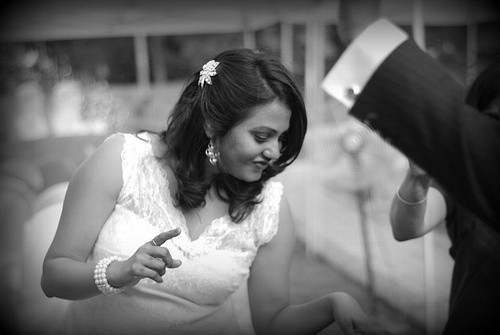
[191,188,225,224]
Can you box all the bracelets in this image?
[94,256,127,296]
[396,185,428,205]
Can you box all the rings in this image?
[151,239,158,247]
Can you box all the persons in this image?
[321,0,500,231]
[390,62,500,335]
[40,49,372,335]
